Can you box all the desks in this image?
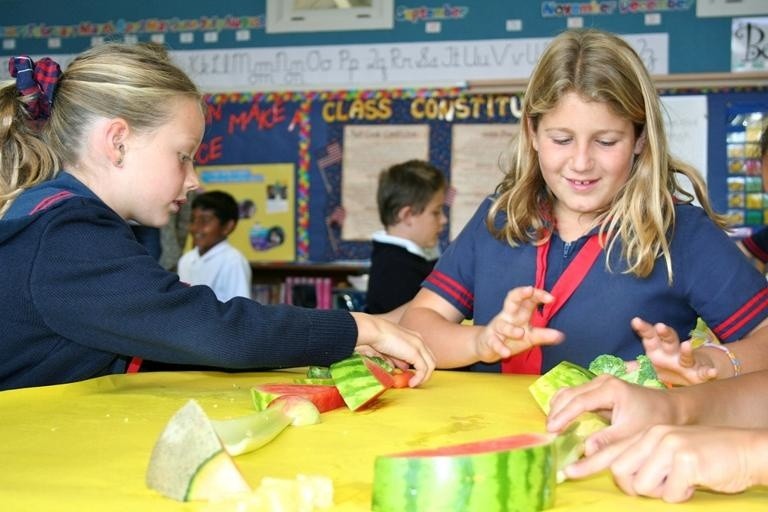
[0,366,768,512]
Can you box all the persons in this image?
[400,26,768,388]
[2,46,437,387]
[546,373,767,503]
[176,191,252,303]
[365,159,446,314]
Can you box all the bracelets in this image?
[701,340,741,378]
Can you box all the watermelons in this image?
[251,354,411,412]
[372,432,559,511]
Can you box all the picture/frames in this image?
[696,0,768,17]
[264,0,394,34]
